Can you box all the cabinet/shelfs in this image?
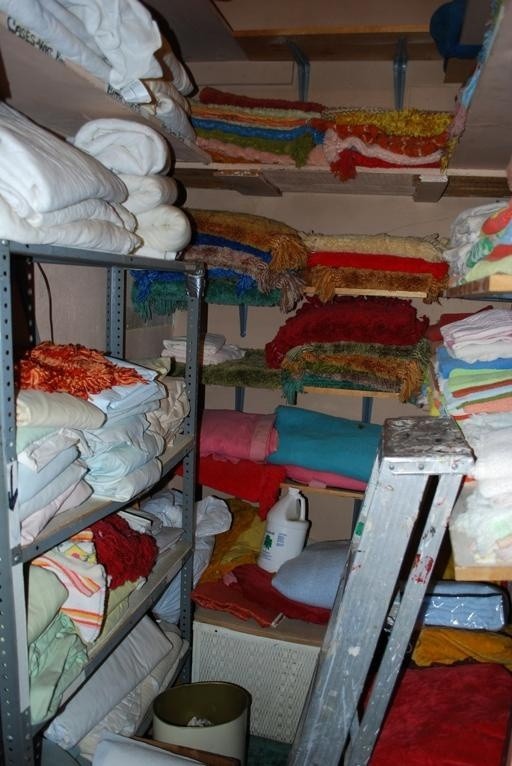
[107,160,510,661]
[2,236,207,766]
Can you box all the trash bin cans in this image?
[152,680,252,765]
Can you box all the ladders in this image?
[290,415,476,765]
